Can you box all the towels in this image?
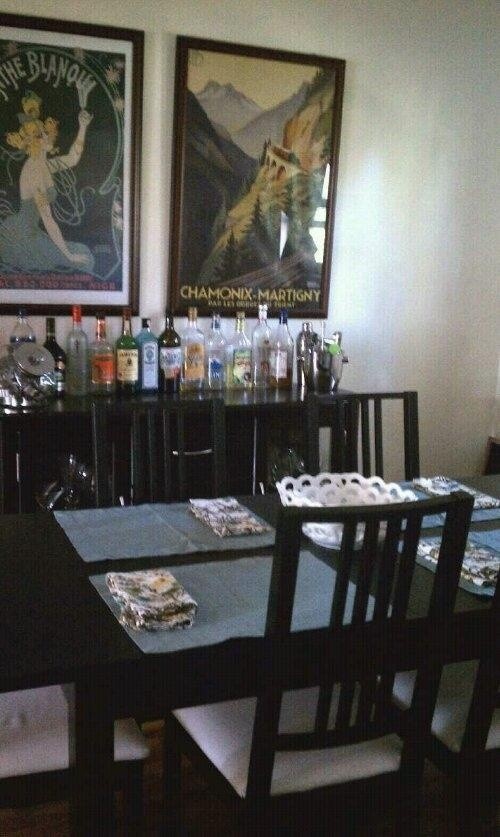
[191,496,275,538]
[414,535,498,592]
[103,566,196,629]
[405,478,496,508]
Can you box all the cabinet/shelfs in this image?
[0,385,360,513]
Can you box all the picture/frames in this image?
[0,11,146,318]
[167,33,347,321]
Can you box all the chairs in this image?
[373,568,500,836]
[304,391,421,486]
[88,397,230,509]
[0,679,153,837]
[160,491,475,837]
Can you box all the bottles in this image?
[207,314,228,390]
[158,314,183,393]
[134,317,159,394]
[267,308,294,387]
[115,307,143,400]
[226,310,253,389]
[87,310,117,395]
[252,302,274,388]
[8,306,36,392]
[179,305,206,394]
[65,304,90,397]
[41,317,67,398]
